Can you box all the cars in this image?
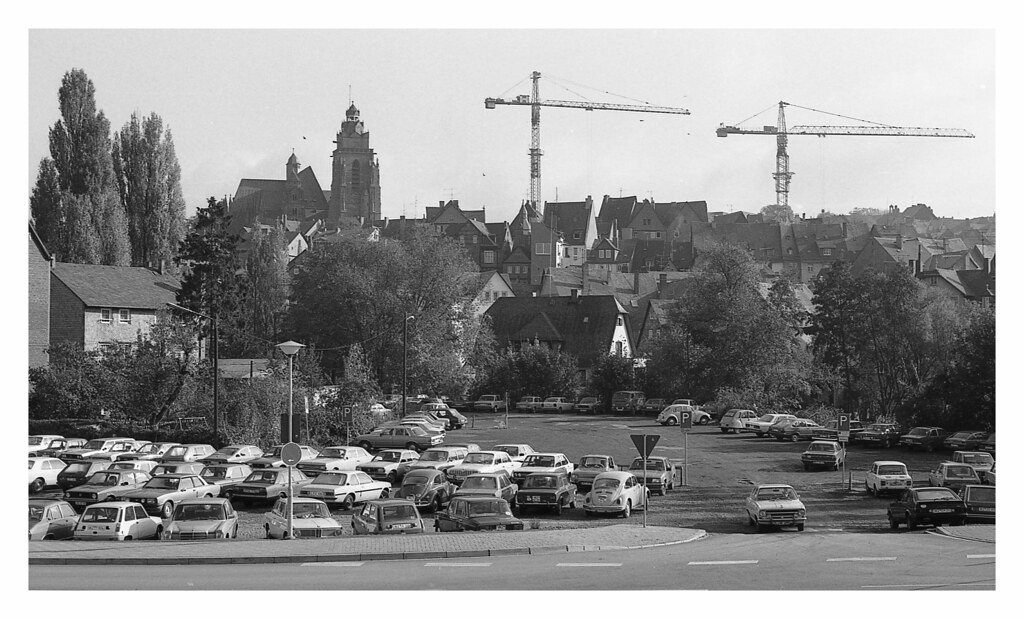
[865,461,913,498]
[352,391,712,454]
[929,462,981,492]
[887,487,968,531]
[27,436,677,543]
[957,485,995,522]
[719,409,995,484]
[744,483,808,532]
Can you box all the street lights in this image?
[276,340,306,538]
[165,301,218,450]
[403,312,415,417]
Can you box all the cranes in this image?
[484,69,692,213]
[715,100,976,206]
